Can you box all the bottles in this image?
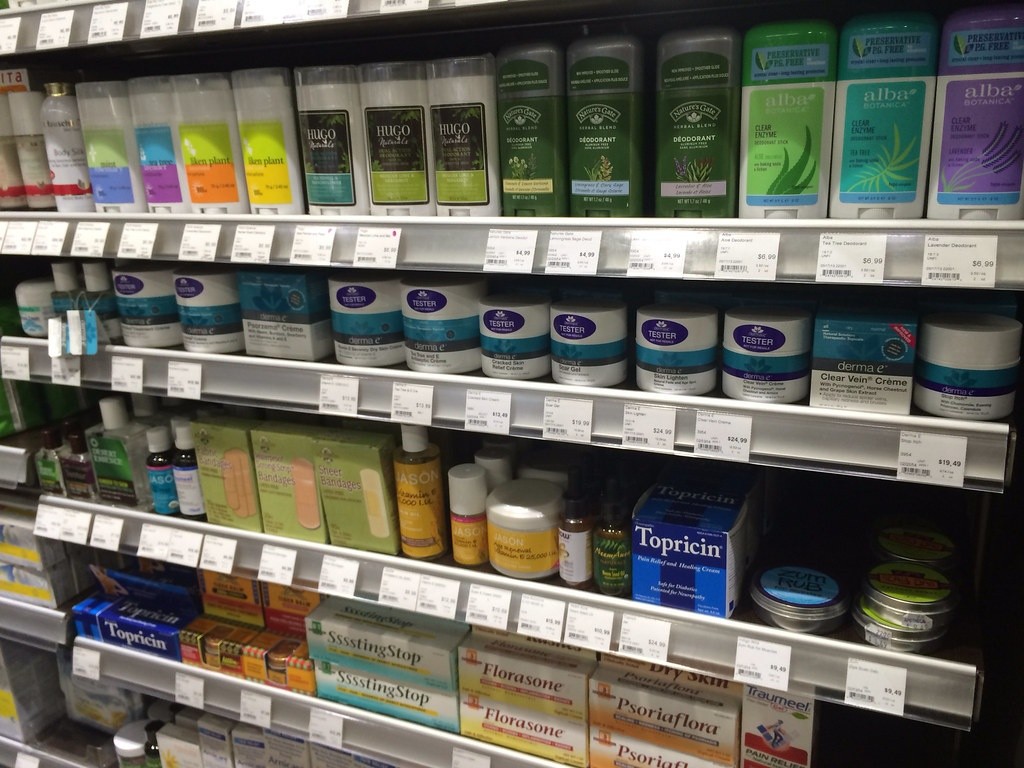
[38,78,97,214]
[85,388,218,521]
[49,259,89,345]
[143,719,164,768]
[33,424,97,502]
[553,490,592,587]
[441,431,514,569]
[390,421,448,561]
[79,259,125,344]
[585,502,634,596]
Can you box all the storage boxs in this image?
[0,262,920,767]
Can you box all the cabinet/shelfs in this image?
[0,1,1024,768]
[0,419,115,768]
